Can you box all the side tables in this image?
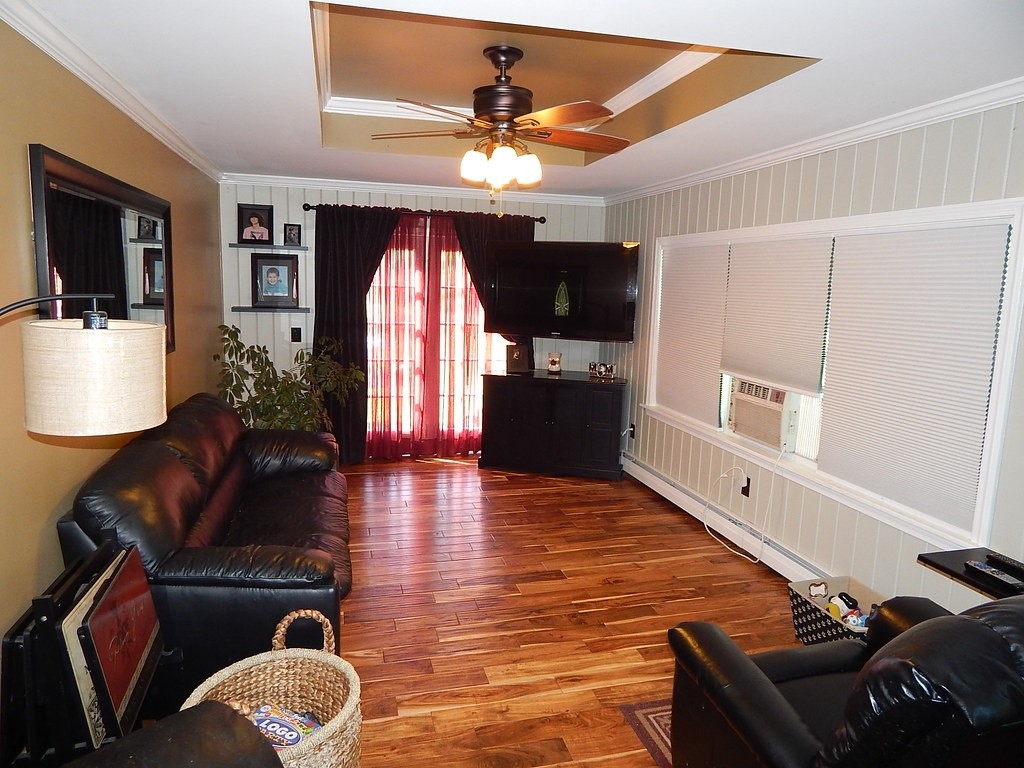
[11,658,230,768]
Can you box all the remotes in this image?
[986,552,1024,574]
[964,559,1024,592]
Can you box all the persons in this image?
[288,226,297,244]
[243,213,268,240]
[263,267,288,296]
[138,216,152,239]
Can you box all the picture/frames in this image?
[137,216,162,240]
[251,253,298,308]
[142,248,164,305]
[237,202,274,244]
[283,223,302,246]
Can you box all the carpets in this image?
[618,699,673,768]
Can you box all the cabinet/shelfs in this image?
[478,368,628,481]
[129,238,164,310]
[227,242,311,314]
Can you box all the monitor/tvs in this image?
[481,240,640,344]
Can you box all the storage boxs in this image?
[788,576,887,646]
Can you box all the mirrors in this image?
[29,143,176,356]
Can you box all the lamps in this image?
[0,293,168,437]
[461,128,543,220]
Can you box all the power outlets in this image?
[291,328,302,343]
[629,423,635,439]
[740,477,750,497]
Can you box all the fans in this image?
[371,47,630,154]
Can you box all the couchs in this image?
[666,594,1024,768]
[55,393,350,659]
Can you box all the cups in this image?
[549,353,562,371]
